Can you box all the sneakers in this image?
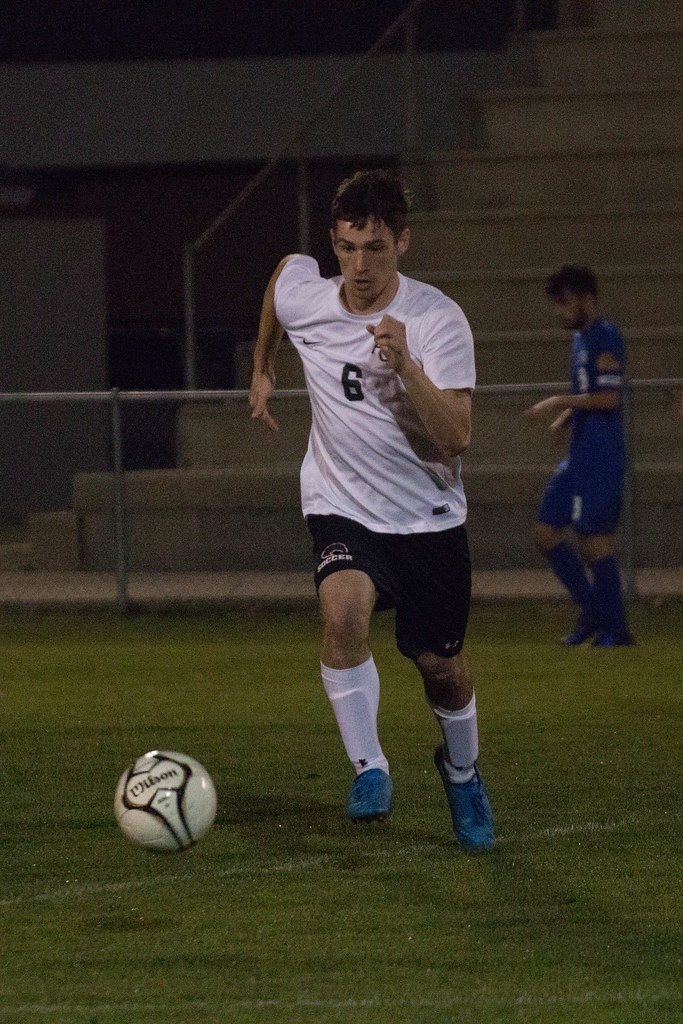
[347,769,391,823]
[434,745,494,849]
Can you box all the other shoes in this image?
[561,622,595,645]
[593,631,631,647]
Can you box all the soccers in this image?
[112,749,219,854]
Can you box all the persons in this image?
[520,260,640,649]
[246,168,508,856]
